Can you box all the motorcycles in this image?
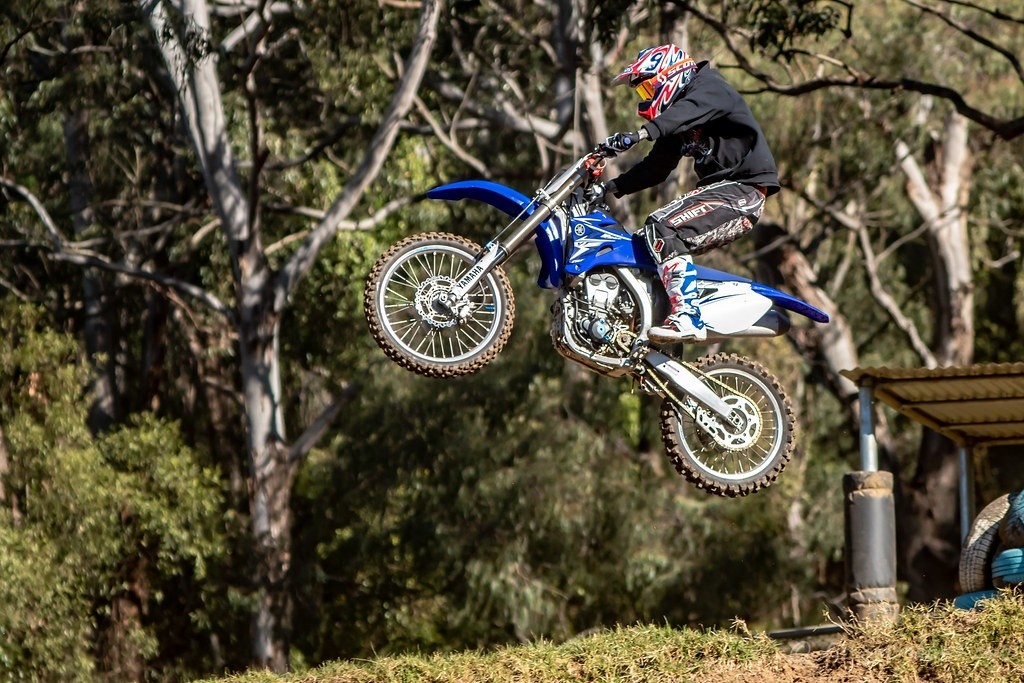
[364,132,830,499]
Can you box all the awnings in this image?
[845,356,1024,442]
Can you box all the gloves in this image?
[606,131,640,153]
[587,182,608,205]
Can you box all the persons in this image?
[585,43,781,351]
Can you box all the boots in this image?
[647,253,707,343]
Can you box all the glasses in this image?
[635,79,657,101]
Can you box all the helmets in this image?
[611,44,698,120]
